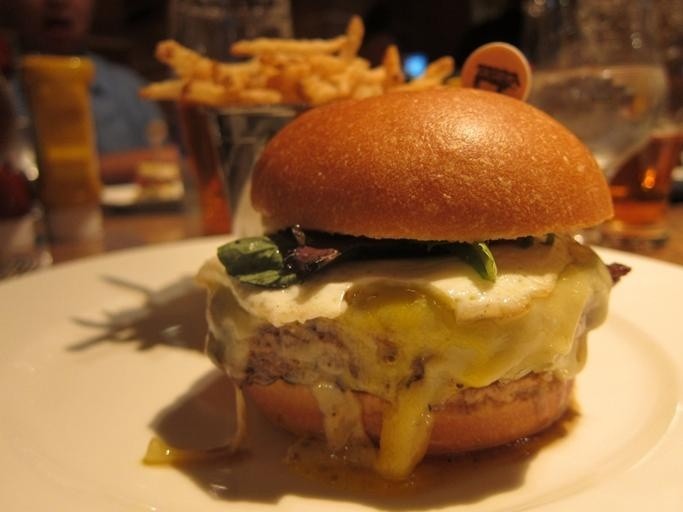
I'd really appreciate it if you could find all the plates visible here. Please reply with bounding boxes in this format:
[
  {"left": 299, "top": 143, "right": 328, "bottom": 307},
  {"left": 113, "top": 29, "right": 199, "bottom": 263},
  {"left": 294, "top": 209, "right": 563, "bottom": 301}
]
[
  {"left": 99, "top": 182, "right": 186, "bottom": 213},
  {"left": 1, "top": 235, "right": 681, "bottom": 510}
]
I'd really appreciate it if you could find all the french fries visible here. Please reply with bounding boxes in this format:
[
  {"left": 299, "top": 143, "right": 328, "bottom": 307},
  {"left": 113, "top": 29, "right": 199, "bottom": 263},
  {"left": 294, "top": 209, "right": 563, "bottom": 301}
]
[{"left": 138, "top": 14, "right": 456, "bottom": 108}]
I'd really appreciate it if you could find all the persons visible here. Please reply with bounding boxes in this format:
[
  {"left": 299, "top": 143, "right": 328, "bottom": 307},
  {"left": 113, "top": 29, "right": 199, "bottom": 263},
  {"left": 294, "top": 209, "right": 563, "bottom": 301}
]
[{"left": 7, "top": 0, "right": 167, "bottom": 151}]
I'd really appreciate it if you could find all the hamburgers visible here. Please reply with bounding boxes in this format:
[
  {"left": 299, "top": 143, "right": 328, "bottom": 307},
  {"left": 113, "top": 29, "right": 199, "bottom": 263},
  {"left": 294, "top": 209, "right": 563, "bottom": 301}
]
[{"left": 141, "top": 85, "right": 632, "bottom": 483}]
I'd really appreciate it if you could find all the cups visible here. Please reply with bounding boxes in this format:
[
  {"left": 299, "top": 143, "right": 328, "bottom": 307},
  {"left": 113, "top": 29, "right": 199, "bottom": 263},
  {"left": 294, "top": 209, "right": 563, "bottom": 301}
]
[{"left": 525, "top": 1, "right": 683, "bottom": 247}]
